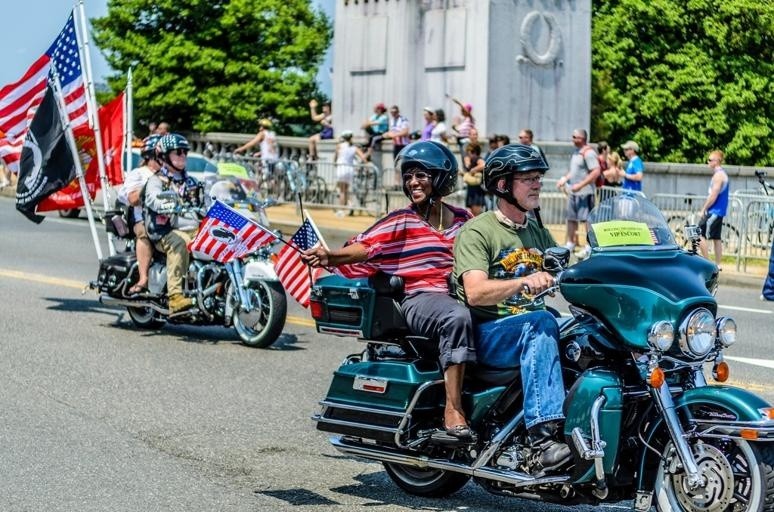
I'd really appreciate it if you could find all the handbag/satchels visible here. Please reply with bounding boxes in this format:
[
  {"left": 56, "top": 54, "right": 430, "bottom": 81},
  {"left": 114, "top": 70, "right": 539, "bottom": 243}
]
[{"left": 462, "top": 171, "right": 484, "bottom": 188}]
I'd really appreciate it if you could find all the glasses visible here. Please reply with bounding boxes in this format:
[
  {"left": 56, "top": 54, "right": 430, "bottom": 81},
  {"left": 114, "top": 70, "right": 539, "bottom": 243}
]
[
  {"left": 169, "top": 148, "right": 189, "bottom": 156},
  {"left": 400, "top": 172, "right": 433, "bottom": 184},
  {"left": 708, "top": 158, "right": 719, "bottom": 162},
  {"left": 506, "top": 173, "right": 546, "bottom": 185}
]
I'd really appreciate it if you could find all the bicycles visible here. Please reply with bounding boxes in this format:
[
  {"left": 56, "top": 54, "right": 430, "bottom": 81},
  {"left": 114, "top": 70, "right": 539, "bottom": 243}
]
[
  {"left": 659, "top": 170, "right": 774, "bottom": 257},
  {"left": 204, "top": 140, "right": 390, "bottom": 219}
]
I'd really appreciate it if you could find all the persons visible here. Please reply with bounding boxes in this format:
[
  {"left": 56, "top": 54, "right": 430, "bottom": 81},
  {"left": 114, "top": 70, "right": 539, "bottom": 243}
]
[
  {"left": 556, "top": 128, "right": 644, "bottom": 255},
  {"left": 332, "top": 132, "right": 369, "bottom": 217},
  {"left": 122, "top": 134, "right": 169, "bottom": 294},
  {"left": 697, "top": 148, "right": 730, "bottom": 271},
  {"left": 452, "top": 142, "right": 614, "bottom": 478},
  {"left": 360, "top": 94, "right": 476, "bottom": 189},
  {"left": 297, "top": 139, "right": 479, "bottom": 442},
  {"left": 131, "top": 121, "right": 171, "bottom": 147},
  {"left": 762, "top": 219, "right": 774, "bottom": 302},
  {"left": 459, "top": 128, "right": 542, "bottom": 224},
  {"left": 138, "top": 131, "right": 209, "bottom": 315},
  {"left": 307, "top": 99, "right": 334, "bottom": 161},
  {"left": 232, "top": 117, "right": 279, "bottom": 207}
]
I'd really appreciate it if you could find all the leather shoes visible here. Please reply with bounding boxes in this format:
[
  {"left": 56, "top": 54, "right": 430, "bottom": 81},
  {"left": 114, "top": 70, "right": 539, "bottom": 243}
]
[{"left": 441, "top": 415, "right": 472, "bottom": 438}]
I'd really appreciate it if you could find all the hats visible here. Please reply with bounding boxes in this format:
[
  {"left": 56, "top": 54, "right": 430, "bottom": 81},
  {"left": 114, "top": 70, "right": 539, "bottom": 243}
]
[
  {"left": 620, "top": 140, "right": 640, "bottom": 153},
  {"left": 453, "top": 97, "right": 475, "bottom": 119},
  {"left": 423, "top": 105, "right": 434, "bottom": 115}
]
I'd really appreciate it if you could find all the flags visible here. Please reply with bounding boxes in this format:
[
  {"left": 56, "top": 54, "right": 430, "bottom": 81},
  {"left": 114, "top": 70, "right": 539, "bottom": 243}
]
[
  {"left": 272, "top": 218, "right": 332, "bottom": 308},
  {"left": 0, "top": 1, "right": 128, "bottom": 225},
  {"left": 185, "top": 199, "right": 277, "bottom": 265}
]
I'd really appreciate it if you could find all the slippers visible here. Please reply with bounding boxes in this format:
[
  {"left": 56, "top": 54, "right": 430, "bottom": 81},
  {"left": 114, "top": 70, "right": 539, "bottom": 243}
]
[{"left": 129, "top": 282, "right": 148, "bottom": 294}]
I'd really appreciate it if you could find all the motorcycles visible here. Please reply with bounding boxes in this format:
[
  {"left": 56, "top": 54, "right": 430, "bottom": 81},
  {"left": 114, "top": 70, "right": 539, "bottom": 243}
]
[
  {"left": 82, "top": 151, "right": 288, "bottom": 349},
  {"left": 306, "top": 196, "right": 774, "bottom": 511}
]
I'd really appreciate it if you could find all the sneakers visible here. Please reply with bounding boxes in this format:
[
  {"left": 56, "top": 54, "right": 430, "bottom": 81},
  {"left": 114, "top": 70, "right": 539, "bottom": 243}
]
[
  {"left": 169, "top": 291, "right": 195, "bottom": 315},
  {"left": 334, "top": 208, "right": 357, "bottom": 218}
]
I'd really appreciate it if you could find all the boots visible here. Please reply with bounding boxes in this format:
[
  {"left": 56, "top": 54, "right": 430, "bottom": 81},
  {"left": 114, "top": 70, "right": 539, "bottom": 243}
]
[{"left": 523, "top": 417, "right": 587, "bottom": 481}]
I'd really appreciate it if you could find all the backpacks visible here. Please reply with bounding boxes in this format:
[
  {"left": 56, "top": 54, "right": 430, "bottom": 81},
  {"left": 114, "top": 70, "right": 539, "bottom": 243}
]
[{"left": 583, "top": 145, "right": 606, "bottom": 188}]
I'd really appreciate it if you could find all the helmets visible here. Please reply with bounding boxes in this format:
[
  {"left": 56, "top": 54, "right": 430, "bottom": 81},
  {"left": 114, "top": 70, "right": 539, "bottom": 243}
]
[
  {"left": 139, "top": 132, "right": 164, "bottom": 156},
  {"left": 482, "top": 141, "right": 551, "bottom": 190},
  {"left": 393, "top": 139, "right": 461, "bottom": 199},
  {"left": 257, "top": 117, "right": 274, "bottom": 129},
  {"left": 154, "top": 131, "right": 191, "bottom": 157},
  {"left": 340, "top": 130, "right": 354, "bottom": 141}
]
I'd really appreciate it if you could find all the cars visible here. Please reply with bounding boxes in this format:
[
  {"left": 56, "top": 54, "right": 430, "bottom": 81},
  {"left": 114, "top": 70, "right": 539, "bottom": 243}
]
[{"left": 58, "top": 145, "right": 217, "bottom": 220}]
